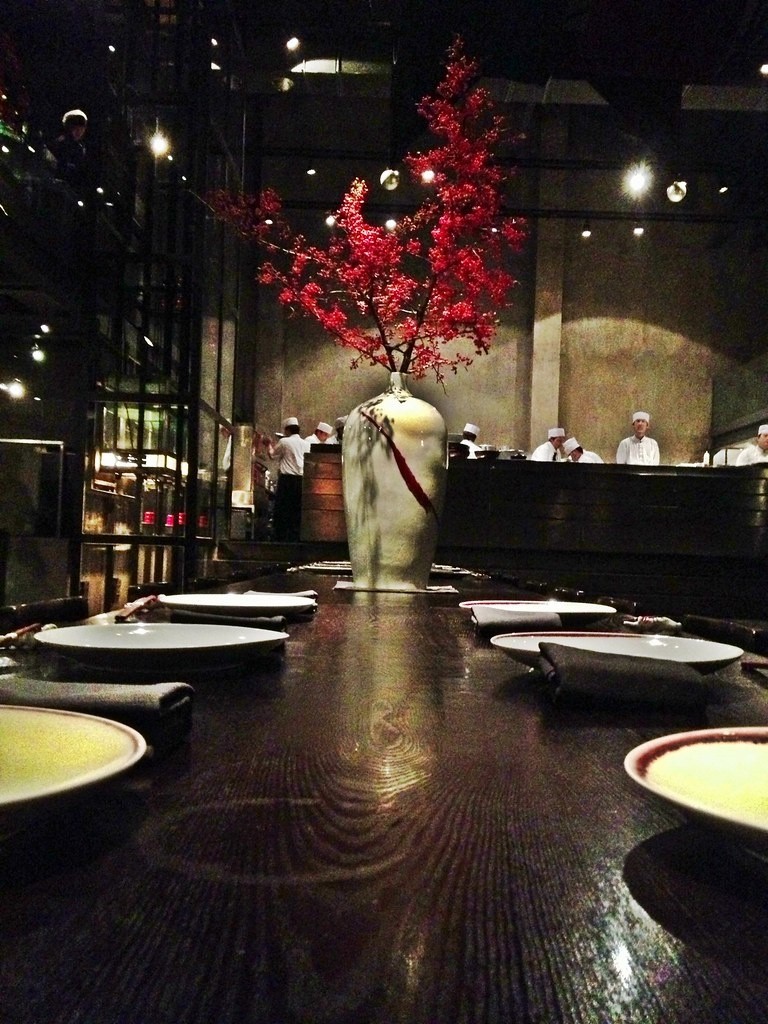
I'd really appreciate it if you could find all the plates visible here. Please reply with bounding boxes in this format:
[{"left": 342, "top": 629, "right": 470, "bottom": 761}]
[
  {"left": 430, "top": 563, "right": 470, "bottom": 575},
  {"left": 34, "top": 623, "right": 290, "bottom": 665},
  {"left": 303, "top": 559, "right": 351, "bottom": 571},
  {"left": 160, "top": 594, "right": 315, "bottom": 616},
  {"left": 0, "top": 703, "right": 147, "bottom": 804},
  {"left": 623, "top": 726, "right": 768, "bottom": 858},
  {"left": 490, "top": 632, "right": 744, "bottom": 678},
  {"left": 458, "top": 600, "right": 617, "bottom": 625}
]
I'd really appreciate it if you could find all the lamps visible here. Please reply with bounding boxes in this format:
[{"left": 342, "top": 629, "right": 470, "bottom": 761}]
[{"left": 581, "top": 218, "right": 592, "bottom": 238}]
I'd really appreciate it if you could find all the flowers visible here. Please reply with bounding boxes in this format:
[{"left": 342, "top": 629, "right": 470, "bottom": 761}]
[{"left": 208, "top": 35, "right": 529, "bottom": 398}]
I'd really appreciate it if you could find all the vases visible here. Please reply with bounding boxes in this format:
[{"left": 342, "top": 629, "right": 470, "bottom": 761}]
[{"left": 342, "top": 371, "right": 448, "bottom": 590}]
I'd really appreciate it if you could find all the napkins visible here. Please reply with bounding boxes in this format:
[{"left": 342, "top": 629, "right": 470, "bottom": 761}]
[
  {"left": 0, "top": 673, "right": 194, "bottom": 708},
  {"left": 537, "top": 642, "right": 712, "bottom": 705},
  {"left": 245, "top": 589, "right": 318, "bottom": 599},
  {"left": 469, "top": 606, "right": 562, "bottom": 636},
  {"left": 173, "top": 608, "right": 286, "bottom": 624}
]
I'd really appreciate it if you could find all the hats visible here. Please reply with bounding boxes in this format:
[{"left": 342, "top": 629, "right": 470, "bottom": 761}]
[
  {"left": 317, "top": 422, "right": 333, "bottom": 434},
  {"left": 334, "top": 415, "right": 348, "bottom": 428},
  {"left": 219, "top": 418, "right": 231, "bottom": 429},
  {"left": 547, "top": 428, "right": 565, "bottom": 440},
  {"left": 633, "top": 411, "right": 650, "bottom": 423},
  {"left": 281, "top": 417, "right": 299, "bottom": 426},
  {"left": 757, "top": 425, "right": 768, "bottom": 435},
  {"left": 561, "top": 437, "right": 580, "bottom": 455},
  {"left": 463, "top": 423, "right": 480, "bottom": 437}
]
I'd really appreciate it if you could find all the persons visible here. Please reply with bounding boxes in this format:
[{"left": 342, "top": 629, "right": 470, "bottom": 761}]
[
  {"left": 460, "top": 423, "right": 484, "bottom": 459},
  {"left": 531, "top": 428, "right": 565, "bottom": 462},
  {"left": 562, "top": 437, "right": 604, "bottom": 463},
  {"left": 266, "top": 417, "right": 311, "bottom": 541},
  {"left": 304, "top": 417, "right": 346, "bottom": 445},
  {"left": 616, "top": 412, "right": 660, "bottom": 465},
  {"left": 735, "top": 425, "right": 768, "bottom": 466},
  {"left": 218, "top": 418, "right": 232, "bottom": 518}
]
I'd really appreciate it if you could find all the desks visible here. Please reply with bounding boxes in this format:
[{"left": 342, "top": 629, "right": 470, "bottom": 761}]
[{"left": 0, "top": 558, "right": 768, "bottom": 1023}]
